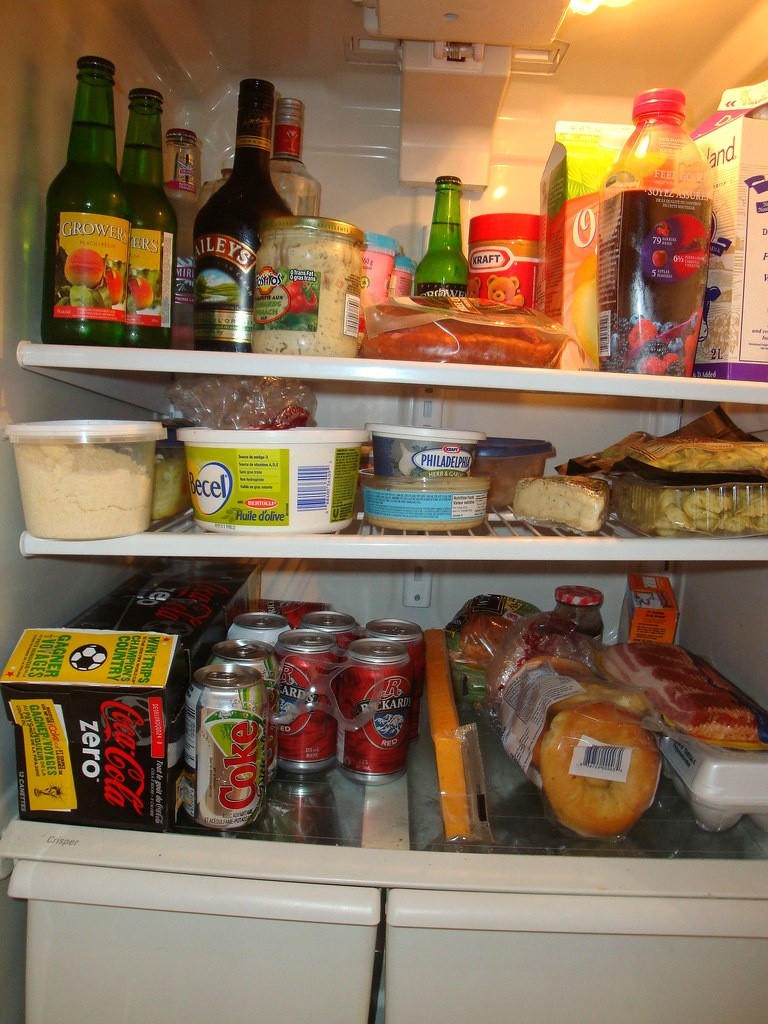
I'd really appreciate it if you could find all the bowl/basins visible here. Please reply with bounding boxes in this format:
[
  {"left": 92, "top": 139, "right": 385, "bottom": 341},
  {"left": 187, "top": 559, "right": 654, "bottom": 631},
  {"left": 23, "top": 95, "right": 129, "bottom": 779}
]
[{"left": 5, "top": 417, "right": 556, "bottom": 541}]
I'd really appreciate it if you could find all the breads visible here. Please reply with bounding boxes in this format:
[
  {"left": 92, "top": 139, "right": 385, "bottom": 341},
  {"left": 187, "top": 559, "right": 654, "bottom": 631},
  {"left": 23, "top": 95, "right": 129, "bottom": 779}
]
[
  {"left": 455, "top": 613, "right": 663, "bottom": 838},
  {"left": 511, "top": 476, "right": 610, "bottom": 531}
]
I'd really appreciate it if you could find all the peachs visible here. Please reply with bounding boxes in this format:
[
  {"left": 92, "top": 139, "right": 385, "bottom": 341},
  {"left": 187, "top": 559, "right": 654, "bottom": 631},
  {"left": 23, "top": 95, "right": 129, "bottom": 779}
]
[{"left": 65, "top": 248, "right": 154, "bottom": 310}]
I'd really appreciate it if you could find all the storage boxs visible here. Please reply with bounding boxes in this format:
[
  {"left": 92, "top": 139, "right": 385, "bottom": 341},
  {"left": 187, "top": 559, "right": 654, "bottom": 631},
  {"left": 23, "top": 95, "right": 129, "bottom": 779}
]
[
  {"left": 617, "top": 572, "right": 679, "bottom": 644},
  {"left": 0, "top": 560, "right": 265, "bottom": 832}
]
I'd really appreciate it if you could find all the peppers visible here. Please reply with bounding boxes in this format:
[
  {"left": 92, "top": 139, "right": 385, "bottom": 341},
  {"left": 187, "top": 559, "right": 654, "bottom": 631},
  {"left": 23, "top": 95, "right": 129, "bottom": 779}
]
[{"left": 276, "top": 269, "right": 318, "bottom": 312}]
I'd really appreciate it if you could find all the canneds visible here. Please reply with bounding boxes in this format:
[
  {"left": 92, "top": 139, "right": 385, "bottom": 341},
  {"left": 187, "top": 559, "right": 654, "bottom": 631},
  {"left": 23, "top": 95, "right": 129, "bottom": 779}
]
[
  {"left": 183, "top": 599, "right": 424, "bottom": 830},
  {"left": 466, "top": 211, "right": 542, "bottom": 305},
  {"left": 549, "top": 586, "right": 605, "bottom": 643},
  {"left": 250, "top": 215, "right": 366, "bottom": 362}
]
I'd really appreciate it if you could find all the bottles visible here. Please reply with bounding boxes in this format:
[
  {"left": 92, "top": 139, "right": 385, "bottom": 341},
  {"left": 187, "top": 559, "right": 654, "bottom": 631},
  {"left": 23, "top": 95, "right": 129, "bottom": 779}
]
[
  {"left": 41, "top": 54, "right": 130, "bottom": 346},
  {"left": 161, "top": 127, "right": 200, "bottom": 350},
  {"left": 552, "top": 584, "right": 604, "bottom": 645},
  {"left": 193, "top": 78, "right": 292, "bottom": 353},
  {"left": 415, "top": 175, "right": 469, "bottom": 297},
  {"left": 270, "top": 96, "right": 322, "bottom": 216},
  {"left": 598, "top": 87, "right": 713, "bottom": 378},
  {"left": 120, "top": 87, "right": 179, "bottom": 349}
]
